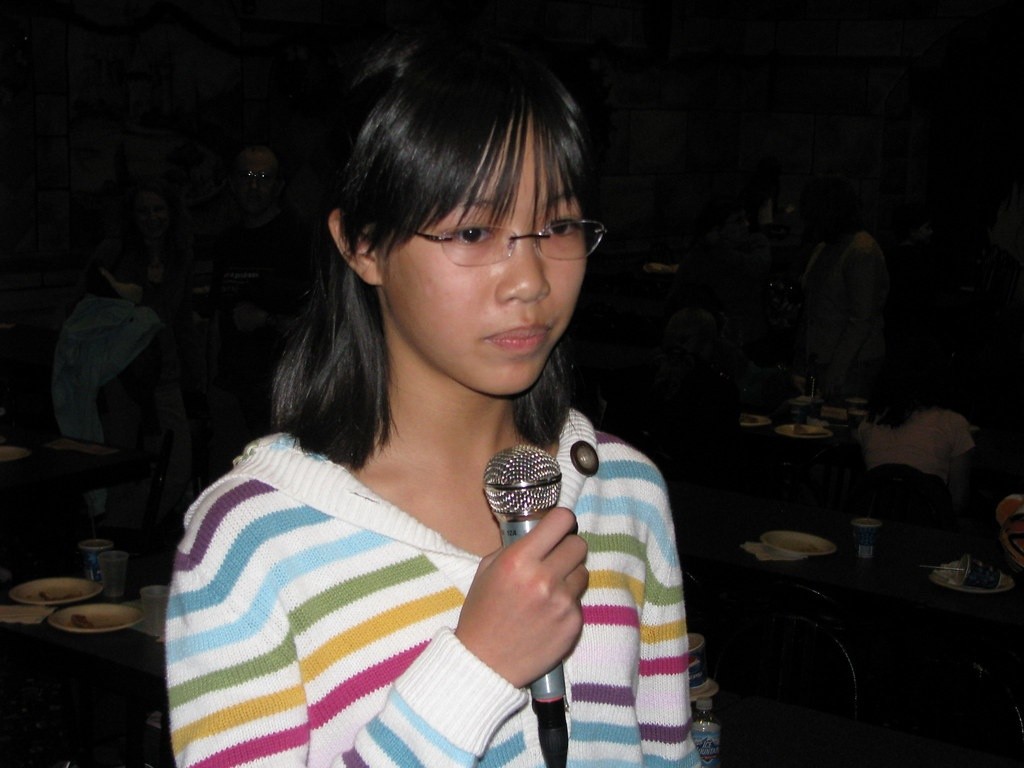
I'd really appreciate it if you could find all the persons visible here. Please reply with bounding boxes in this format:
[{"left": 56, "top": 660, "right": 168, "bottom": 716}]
[
  {"left": 786, "top": 172, "right": 890, "bottom": 405},
  {"left": 209, "top": 146, "right": 309, "bottom": 485},
  {"left": 859, "top": 341, "right": 977, "bottom": 489},
  {"left": 598, "top": 305, "right": 752, "bottom": 490},
  {"left": 165, "top": 39, "right": 698, "bottom": 768},
  {"left": 892, "top": 200, "right": 947, "bottom": 294},
  {"left": 87, "top": 177, "right": 199, "bottom": 553},
  {"left": 704, "top": 189, "right": 779, "bottom": 305}
]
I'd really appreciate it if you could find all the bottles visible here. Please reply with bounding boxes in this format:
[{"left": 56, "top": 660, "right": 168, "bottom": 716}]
[{"left": 691, "top": 697, "right": 721, "bottom": 768}]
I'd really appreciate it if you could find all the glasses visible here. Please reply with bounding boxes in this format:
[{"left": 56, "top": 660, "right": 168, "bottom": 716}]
[
  {"left": 235, "top": 168, "right": 271, "bottom": 188},
  {"left": 411, "top": 215, "right": 609, "bottom": 266},
  {"left": 725, "top": 214, "right": 746, "bottom": 226}
]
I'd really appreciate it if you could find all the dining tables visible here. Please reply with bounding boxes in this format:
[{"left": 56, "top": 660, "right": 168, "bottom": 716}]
[{"left": 2, "top": 261, "right": 1024, "bottom": 768}]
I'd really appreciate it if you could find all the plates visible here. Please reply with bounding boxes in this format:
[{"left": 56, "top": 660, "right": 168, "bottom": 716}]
[
  {"left": 689, "top": 677, "right": 718, "bottom": 702},
  {"left": 761, "top": 530, "right": 837, "bottom": 555},
  {"left": 9, "top": 577, "right": 103, "bottom": 605},
  {"left": 929, "top": 563, "right": 1015, "bottom": 593},
  {"left": 774, "top": 424, "right": 833, "bottom": 439},
  {"left": 820, "top": 406, "right": 846, "bottom": 421},
  {"left": 740, "top": 414, "right": 772, "bottom": 426},
  {"left": 0, "top": 446, "right": 31, "bottom": 462},
  {"left": 47, "top": 603, "right": 143, "bottom": 634}
]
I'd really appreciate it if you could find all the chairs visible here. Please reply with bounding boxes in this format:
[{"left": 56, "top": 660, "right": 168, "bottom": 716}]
[
  {"left": 905, "top": 653, "right": 1024, "bottom": 762},
  {"left": 858, "top": 464, "right": 957, "bottom": 533},
  {"left": 713, "top": 614, "right": 859, "bottom": 722}
]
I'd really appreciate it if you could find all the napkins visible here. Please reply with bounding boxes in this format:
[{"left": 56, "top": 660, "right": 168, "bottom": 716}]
[{"left": 739, "top": 539, "right": 808, "bottom": 562}]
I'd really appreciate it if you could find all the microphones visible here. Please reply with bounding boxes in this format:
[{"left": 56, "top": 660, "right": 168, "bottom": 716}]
[{"left": 482, "top": 442, "right": 569, "bottom": 767}]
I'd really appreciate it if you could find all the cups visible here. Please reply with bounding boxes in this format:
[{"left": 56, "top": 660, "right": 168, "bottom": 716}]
[
  {"left": 851, "top": 518, "right": 881, "bottom": 557},
  {"left": 847, "top": 398, "right": 869, "bottom": 431},
  {"left": 79, "top": 538, "right": 113, "bottom": 582},
  {"left": 687, "top": 632, "right": 708, "bottom": 689},
  {"left": 789, "top": 401, "right": 808, "bottom": 424},
  {"left": 809, "top": 399, "right": 824, "bottom": 418},
  {"left": 955, "top": 554, "right": 1002, "bottom": 591},
  {"left": 98, "top": 550, "right": 129, "bottom": 598},
  {"left": 139, "top": 585, "right": 168, "bottom": 638}
]
